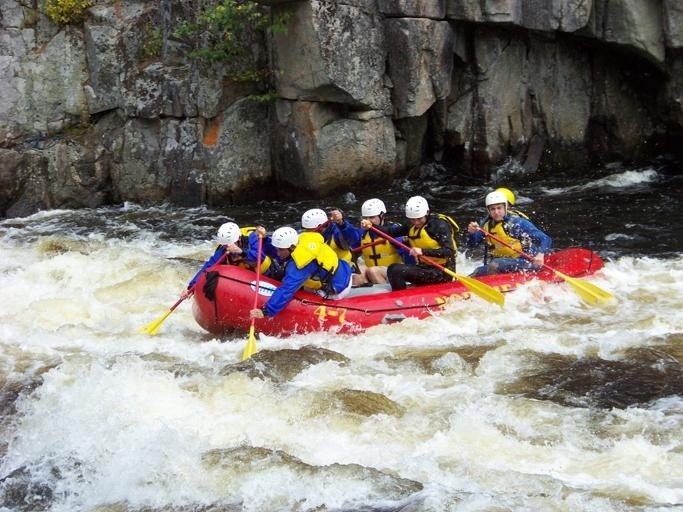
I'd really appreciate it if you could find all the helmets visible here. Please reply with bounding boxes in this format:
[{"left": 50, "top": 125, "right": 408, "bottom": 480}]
[
  {"left": 405, "top": 196, "right": 429, "bottom": 219},
  {"left": 495, "top": 188, "right": 515, "bottom": 206},
  {"left": 218, "top": 222, "right": 240, "bottom": 245},
  {"left": 361, "top": 199, "right": 386, "bottom": 217},
  {"left": 486, "top": 192, "right": 507, "bottom": 210},
  {"left": 271, "top": 227, "right": 298, "bottom": 248},
  {"left": 302, "top": 209, "right": 328, "bottom": 228}
]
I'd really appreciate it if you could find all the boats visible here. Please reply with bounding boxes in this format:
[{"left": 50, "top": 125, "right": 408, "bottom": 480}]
[{"left": 193, "top": 250, "right": 604, "bottom": 338}]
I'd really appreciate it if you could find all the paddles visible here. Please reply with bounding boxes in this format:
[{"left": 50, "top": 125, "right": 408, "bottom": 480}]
[
  {"left": 363, "top": 222, "right": 505, "bottom": 308},
  {"left": 243, "top": 226, "right": 265, "bottom": 360},
  {"left": 468, "top": 223, "right": 614, "bottom": 306},
  {"left": 139, "top": 250, "right": 231, "bottom": 335}
]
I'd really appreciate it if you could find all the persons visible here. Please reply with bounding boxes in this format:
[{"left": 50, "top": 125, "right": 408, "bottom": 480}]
[
  {"left": 360, "top": 195, "right": 459, "bottom": 293},
  {"left": 249, "top": 227, "right": 353, "bottom": 318},
  {"left": 301, "top": 208, "right": 357, "bottom": 273},
  {"left": 179, "top": 222, "right": 287, "bottom": 302},
  {"left": 496, "top": 187, "right": 532, "bottom": 223},
  {"left": 463, "top": 191, "right": 552, "bottom": 278},
  {"left": 330, "top": 199, "right": 416, "bottom": 287}
]
